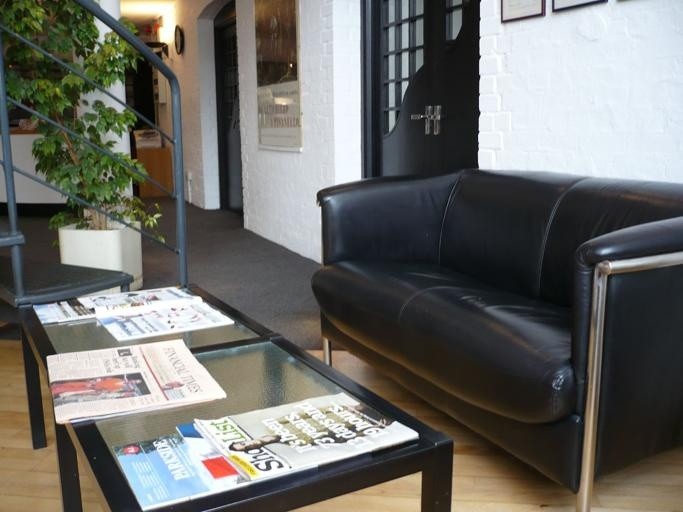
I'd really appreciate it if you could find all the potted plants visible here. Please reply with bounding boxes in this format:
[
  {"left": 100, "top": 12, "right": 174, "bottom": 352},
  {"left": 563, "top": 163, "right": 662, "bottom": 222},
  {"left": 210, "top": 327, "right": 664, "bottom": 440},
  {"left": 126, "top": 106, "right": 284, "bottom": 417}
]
[{"left": 0, "top": 0, "right": 166, "bottom": 294}]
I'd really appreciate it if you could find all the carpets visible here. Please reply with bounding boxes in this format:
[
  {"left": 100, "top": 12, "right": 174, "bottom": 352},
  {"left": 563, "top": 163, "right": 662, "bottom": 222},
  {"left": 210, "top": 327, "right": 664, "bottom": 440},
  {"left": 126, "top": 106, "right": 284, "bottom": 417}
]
[{"left": 0, "top": 339, "right": 683, "bottom": 512}]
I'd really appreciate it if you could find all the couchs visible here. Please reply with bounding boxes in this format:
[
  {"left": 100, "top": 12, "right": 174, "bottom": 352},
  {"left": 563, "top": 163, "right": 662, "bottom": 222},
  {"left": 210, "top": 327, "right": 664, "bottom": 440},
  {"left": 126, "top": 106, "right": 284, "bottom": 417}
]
[{"left": 311, "top": 168, "right": 683, "bottom": 512}]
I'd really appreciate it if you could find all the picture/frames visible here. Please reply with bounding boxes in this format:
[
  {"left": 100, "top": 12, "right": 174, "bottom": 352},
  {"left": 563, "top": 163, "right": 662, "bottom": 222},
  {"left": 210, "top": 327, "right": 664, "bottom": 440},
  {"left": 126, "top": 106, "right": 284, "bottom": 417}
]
[
  {"left": 552, "top": 0, "right": 608, "bottom": 12},
  {"left": 500, "top": 0, "right": 546, "bottom": 23}
]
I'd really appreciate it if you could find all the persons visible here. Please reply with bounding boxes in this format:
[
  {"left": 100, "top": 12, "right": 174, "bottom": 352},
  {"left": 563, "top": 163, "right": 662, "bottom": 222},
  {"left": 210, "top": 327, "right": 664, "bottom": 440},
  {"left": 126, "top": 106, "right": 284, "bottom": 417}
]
[
  {"left": 50, "top": 374, "right": 143, "bottom": 397},
  {"left": 229, "top": 402, "right": 393, "bottom": 452}
]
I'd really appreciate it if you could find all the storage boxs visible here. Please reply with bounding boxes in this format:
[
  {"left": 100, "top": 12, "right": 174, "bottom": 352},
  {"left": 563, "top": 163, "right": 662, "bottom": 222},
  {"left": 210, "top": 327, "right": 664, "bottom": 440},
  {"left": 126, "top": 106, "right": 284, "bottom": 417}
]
[{"left": 133, "top": 129, "right": 162, "bottom": 149}]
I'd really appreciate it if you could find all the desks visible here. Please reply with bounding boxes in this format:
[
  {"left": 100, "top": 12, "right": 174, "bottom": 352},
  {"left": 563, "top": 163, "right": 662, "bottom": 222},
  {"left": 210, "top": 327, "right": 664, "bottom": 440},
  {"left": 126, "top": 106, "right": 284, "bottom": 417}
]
[{"left": 136, "top": 147, "right": 173, "bottom": 198}]
